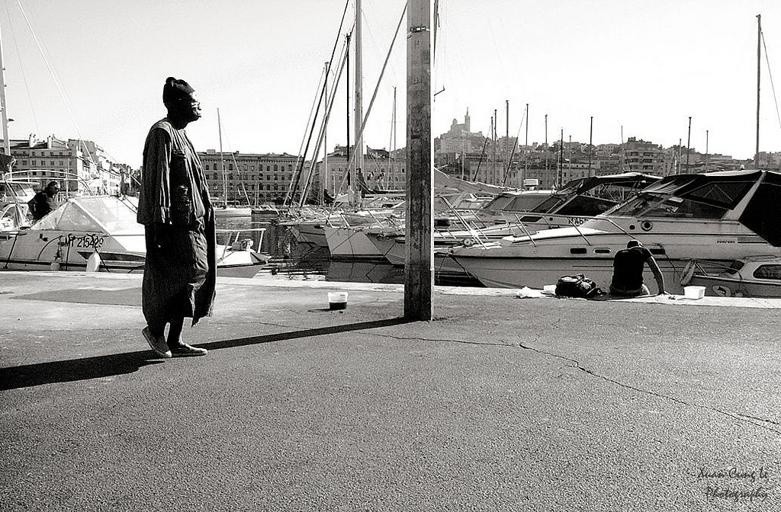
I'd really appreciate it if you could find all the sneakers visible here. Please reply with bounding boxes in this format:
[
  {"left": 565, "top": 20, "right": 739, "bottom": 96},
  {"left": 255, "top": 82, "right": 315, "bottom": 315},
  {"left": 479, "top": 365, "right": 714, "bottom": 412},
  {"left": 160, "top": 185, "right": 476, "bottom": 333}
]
[
  {"left": 142, "top": 326, "right": 172, "bottom": 358},
  {"left": 171, "top": 343, "right": 208, "bottom": 358}
]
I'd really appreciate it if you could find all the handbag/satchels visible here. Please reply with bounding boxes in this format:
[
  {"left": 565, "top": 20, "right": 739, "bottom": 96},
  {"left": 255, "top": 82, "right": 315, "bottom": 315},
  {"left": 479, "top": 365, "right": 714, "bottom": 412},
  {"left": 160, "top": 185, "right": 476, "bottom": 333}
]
[{"left": 555, "top": 273, "right": 608, "bottom": 299}]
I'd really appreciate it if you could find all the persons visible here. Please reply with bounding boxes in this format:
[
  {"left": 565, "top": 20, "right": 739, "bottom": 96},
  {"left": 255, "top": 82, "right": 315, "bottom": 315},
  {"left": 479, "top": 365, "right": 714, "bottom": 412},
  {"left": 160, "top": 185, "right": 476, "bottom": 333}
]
[
  {"left": 367, "top": 171, "right": 385, "bottom": 190},
  {"left": 609, "top": 240, "right": 669, "bottom": 299},
  {"left": 324, "top": 189, "right": 335, "bottom": 203},
  {"left": 28, "top": 181, "right": 61, "bottom": 224},
  {"left": 136, "top": 77, "right": 216, "bottom": 358}
]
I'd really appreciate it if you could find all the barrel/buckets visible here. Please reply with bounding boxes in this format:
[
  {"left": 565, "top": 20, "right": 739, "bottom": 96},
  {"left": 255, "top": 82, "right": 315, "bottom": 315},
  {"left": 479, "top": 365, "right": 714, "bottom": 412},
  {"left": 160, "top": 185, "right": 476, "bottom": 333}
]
[{"left": 327, "top": 292, "right": 348, "bottom": 310}]
